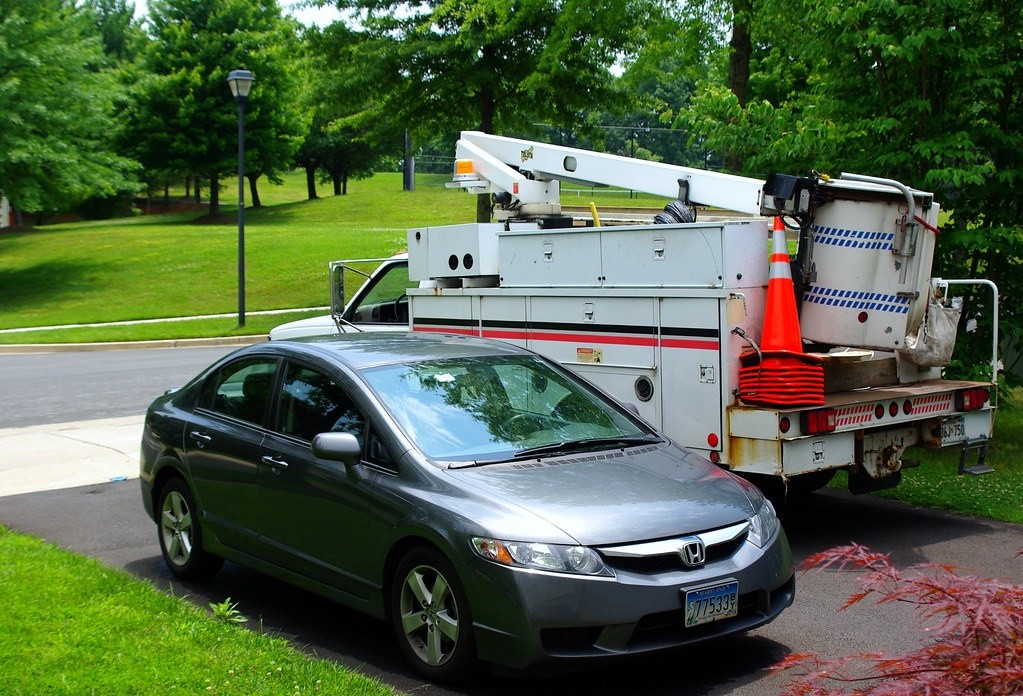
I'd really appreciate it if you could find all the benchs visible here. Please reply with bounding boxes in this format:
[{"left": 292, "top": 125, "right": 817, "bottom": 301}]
[{"left": 225, "top": 372, "right": 274, "bottom": 425}]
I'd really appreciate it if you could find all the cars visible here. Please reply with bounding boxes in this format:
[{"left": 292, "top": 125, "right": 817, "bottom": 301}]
[{"left": 138, "top": 329, "right": 794, "bottom": 690}]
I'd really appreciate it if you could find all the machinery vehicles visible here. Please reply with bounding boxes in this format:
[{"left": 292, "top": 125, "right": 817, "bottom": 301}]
[{"left": 267, "top": 131, "right": 1000, "bottom": 503}]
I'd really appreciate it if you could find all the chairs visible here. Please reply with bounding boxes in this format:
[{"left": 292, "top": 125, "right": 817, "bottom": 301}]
[{"left": 284, "top": 373, "right": 343, "bottom": 441}]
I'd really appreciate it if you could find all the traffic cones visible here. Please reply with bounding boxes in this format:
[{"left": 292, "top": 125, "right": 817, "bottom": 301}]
[{"left": 737, "top": 216, "right": 825, "bottom": 408}]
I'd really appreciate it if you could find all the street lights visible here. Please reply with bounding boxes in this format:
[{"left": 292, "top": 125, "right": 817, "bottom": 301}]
[{"left": 227, "top": 65, "right": 255, "bottom": 329}]
